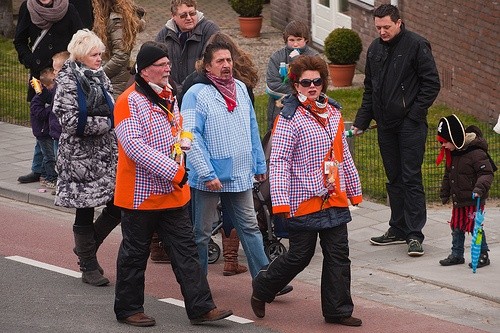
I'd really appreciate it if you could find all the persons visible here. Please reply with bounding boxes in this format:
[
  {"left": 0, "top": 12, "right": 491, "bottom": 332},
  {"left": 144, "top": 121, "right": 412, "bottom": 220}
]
[
  {"left": 113, "top": 39, "right": 233, "bottom": 326},
  {"left": 13, "top": 1, "right": 321, "bottom": 296},
  {"left": 351, "top": 4, "right": 441, "bottom": 256},
  {"left": 434, "top": 114, "right": 498, "bottom": 269},
  {"left": 250, "top": 54, "right": 363, "bottom": 327}
]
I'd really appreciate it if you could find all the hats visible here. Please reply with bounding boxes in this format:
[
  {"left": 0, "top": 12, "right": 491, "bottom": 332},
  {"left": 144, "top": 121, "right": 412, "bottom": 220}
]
[
  {"left": 436, "top": 119, "right": 453, "bottom": 144},
  {"left": 137, "top": 45, "right": 170, "bottom": 74}
]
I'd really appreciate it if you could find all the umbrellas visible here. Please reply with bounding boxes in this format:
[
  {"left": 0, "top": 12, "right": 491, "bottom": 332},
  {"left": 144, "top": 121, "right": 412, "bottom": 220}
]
[{"left": 470, "top": 193, "right": 485, "bottom": 273}]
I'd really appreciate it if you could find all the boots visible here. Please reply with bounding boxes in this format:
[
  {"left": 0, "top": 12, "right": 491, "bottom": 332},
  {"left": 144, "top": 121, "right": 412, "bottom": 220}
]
[
  {"left": 73, "top": 207, "right": 121, "bottom": 275},
  {"left": 220, "top": 227, "right": 247, "bottom": 275},
  {"left": 73, "top": 223, "right": 110, "bottom": 286}
]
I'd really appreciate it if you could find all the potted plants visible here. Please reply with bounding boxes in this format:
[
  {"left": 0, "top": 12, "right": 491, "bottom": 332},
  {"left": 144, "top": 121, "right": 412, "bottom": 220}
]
[
  {"left": 322, "top": 28, "right": 362, "bottom": 86},
  {"left": 229, "top": 0, "right": 264, "bottom": 37}
]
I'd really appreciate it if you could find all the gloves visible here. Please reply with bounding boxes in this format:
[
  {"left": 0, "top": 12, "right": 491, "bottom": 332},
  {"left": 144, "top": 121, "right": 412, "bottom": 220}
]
[
  {"left": 178, "top": 167, "right": 190, "bottom": 188},
  {"left": 92, "top": 104, "right": 111, "bottom": 117}
]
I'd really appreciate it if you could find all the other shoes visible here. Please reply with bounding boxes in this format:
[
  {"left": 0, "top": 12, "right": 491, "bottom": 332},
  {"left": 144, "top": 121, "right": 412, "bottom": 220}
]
[
  {"left": 251, "top": 293, "right": 265, "bottom": 318},
  {"left": 325, "top": 315, "right": 362, "bottom": 326},
  {"left": 190, "top": 307, "right": 233, "bottom": 324},
  {"left": 276, "top": 285, "right": 293, "bottom": 296},
  {"left": 150, "top": 238, "right": 171, "bottom": 261},
  {"left": 117, "top": 312, "right": 155, "bottom": 327},
  {"left": 40, "top": 177, "right": 56, "bottom": 189},
  {"left": 18, "top": 172, "right": 41, "bottom": 183}
]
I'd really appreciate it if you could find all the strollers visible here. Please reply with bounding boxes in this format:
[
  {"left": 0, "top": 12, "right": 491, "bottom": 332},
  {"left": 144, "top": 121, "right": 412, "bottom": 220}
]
[{"left": 206, "top": 177, "right": 287, "bottom": 266}]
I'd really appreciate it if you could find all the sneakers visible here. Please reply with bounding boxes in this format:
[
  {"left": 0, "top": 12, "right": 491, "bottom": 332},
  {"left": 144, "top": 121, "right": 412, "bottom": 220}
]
[
  {"left": 369, "top": 231, "right": 406, "bottom": 245},
  {"left": 407, "top": 239, "right": 424, "bottom": 256},
  {"left": 468, "top": 252, "right": 490, "bottom": 268},
  {"left": 439, "top": 254, "right": 465, "bottom": 266}
]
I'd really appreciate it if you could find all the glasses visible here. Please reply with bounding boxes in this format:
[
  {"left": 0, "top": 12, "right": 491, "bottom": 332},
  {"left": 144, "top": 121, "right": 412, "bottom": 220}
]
[
  {"left": 151, "top": 63, "right": 173, "bottom": 68},
  {"left": 175, "top": 11, "right": 197, "bottom": 19},
  {"left": 296, "top": 78, "right": 323, "bottom": 87}
]
